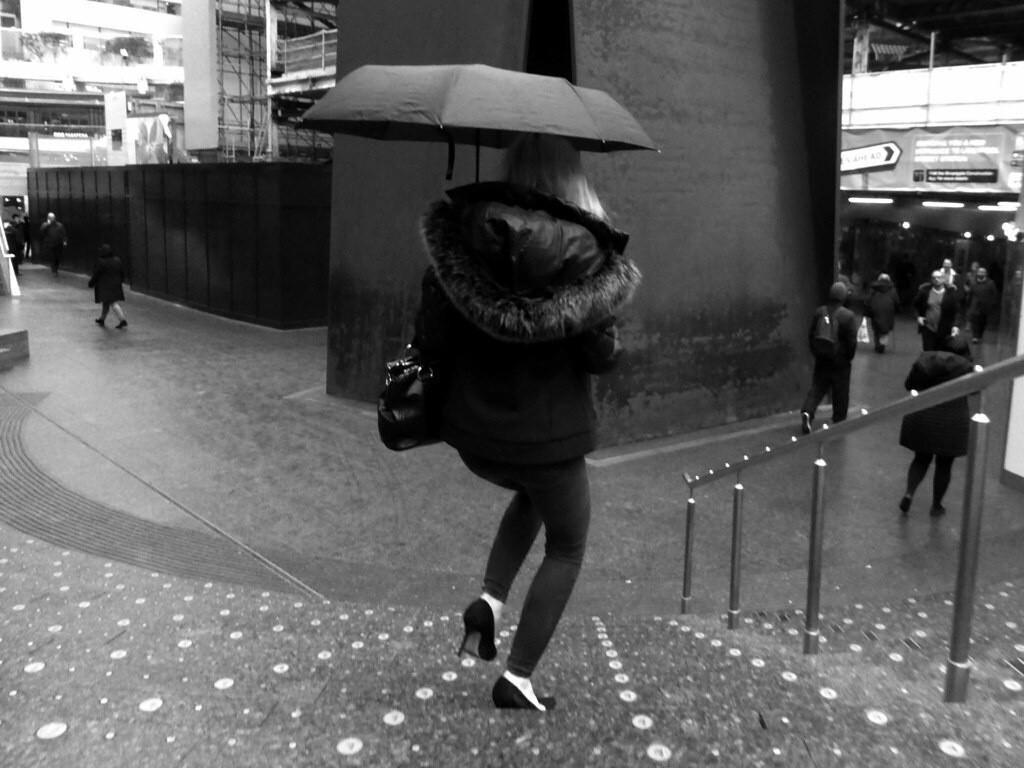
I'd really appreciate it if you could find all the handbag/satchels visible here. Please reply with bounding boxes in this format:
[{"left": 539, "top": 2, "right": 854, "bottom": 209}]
[
  {"left": 377, "top": 353, "right": 443, "bottom": 451},
  {"left": 856, "top": 316, "right": 869, "bottom": 343}
]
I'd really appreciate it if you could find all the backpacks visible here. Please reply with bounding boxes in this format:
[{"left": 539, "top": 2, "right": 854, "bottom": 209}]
[{"left": 812, "top": 305, "right": 846, "bottom": 354}]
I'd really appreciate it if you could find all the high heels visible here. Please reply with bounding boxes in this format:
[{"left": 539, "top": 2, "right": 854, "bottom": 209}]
[
  {"left": 493, "top": 675, "right": 556, "bottom": 712},
  {"left": 458, "top": 598, "right": 497, "bottom": 660}
]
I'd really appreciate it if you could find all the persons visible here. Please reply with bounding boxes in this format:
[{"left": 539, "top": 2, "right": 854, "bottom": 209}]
[
  {"left": 898, "top": 335, "right": 981, "bottom": 517},
  {"left": 86, "top": 245, "right": 128, "bottom": 330},
  {"left": 897, "top": 254, "right": 917, "bottom": 307},
  {"left": 801, "top": 274, "right": 861, "bottom": 434},
  {"left": 4, "top": 213, "right": 32, "bottom": 278},
  {"left": 916, "top": 258, "right": 997, "bottom": 350},
  {"left": 41, "top": 212, "right": 68, "bottom": 277},
  {"left": 416, "top": 134, "right": 642, "bottom": 716},
  {"left": 1005, "top": 232, "right": 1024, "bottom": 339},
  {"left": 864, "top": 272, "right": 901, "bottom": 352}
]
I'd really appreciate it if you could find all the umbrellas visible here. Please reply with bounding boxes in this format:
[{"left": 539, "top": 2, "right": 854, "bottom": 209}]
[{"left": 298, "top": 62, "right": 661, "bottom": 184}]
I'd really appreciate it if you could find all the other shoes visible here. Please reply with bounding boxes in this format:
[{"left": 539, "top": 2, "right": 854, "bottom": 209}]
[
  {"left": 115, "top": 320, "right": 128, "bottom": 328},
  {"left": 802, "top": 412, "right": 811, "bottom": 434},
  {"left": 931, "top": 506, "right": 945, "bottom": 516},
  {"left": 899, "top": 497, "right": 911, "bottom": 512},
  {"left": 96, "top": 319, "right": 105, "bottom": 325}
]
